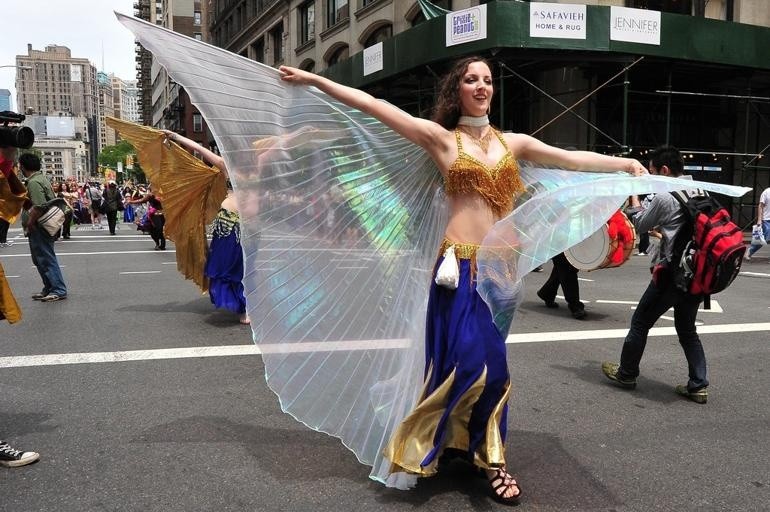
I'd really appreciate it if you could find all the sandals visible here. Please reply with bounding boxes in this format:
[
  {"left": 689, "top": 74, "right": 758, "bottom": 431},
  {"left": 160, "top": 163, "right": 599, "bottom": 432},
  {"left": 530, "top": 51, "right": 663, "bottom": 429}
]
[
  {"left": 572, "top": 306, "right": 585, "bottom": 321},
  {"left": 537, "top": 291, "right": 559, "bottom": 309},
  {"left": 486, "top": 464, "right": 523, "bottom": 502}
]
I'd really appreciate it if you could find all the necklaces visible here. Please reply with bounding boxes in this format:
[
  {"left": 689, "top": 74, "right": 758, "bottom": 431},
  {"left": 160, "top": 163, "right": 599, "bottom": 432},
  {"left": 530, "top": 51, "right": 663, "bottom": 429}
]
[{"left": 458, "top": 126, "right": 492, "bottom": 155}]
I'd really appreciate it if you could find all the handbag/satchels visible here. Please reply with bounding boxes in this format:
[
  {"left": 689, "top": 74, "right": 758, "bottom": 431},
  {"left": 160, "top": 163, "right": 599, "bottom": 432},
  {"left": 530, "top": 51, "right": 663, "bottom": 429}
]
[
  {"left": 29, "top": 181, "right": 66, "bottom": 237},
  {"left": 99, "top": 200, "right": 118, "bottom": 215},
  {"left": 88, "top": 189, "right": 102, "bottom": 210},
  {"left": 752, "top": 223, "right": 767, "bottom": 246}
]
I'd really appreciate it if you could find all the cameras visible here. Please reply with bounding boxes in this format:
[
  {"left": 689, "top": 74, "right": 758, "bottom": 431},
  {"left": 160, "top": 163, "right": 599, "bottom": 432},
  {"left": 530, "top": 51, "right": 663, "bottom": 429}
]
[
  {"left": 603, "top": 145, "right": 650, "bottom": 200},
  {"left": 1, "top": 110, "right": 34, "bottom": 149}
]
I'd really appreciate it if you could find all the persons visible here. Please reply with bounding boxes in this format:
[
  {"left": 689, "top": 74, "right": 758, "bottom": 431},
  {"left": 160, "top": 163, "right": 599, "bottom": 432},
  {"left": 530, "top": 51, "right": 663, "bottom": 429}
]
[
  {"left": 50, "top": 174, "right": 167, "bottom": 252},
  {"left": 533, "top": 266, "right": 544, "bottom": 272},
  {"left": 0, "top": 147, "right": 40, "bottom": 467},
  {"left": 18, "top": 153, "right": 68, "bottom": 303},
  {"left": 537, "top": 252, "right": 589, "bottom": 320},
  {"left": 601, "top": 144, "right": 712, "bottom": 404},
  {"left": 158, "top": 124, "right": 316, "bottom": 325},
  {"left": 279, "top": 55, "right": 649, "bottom": 505},
  {"left": 260, "top": 179, "right": 361, "bottom": 248},
  {"left": 637, "top": 193, "right": 655, "bottom": 256},
  {"left": 743, "top": 187, "right": 770, "bottom": 262}
]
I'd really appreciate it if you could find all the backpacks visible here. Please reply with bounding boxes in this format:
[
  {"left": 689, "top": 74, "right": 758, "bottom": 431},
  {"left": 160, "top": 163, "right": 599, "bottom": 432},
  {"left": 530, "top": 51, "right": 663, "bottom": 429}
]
[{"left": 650, "top": 189, "right": 748, "bottom": 309}]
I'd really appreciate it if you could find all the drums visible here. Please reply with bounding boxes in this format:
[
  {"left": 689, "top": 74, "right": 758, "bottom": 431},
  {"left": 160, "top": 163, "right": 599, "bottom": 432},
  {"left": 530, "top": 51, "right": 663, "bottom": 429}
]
[{"left": 565, "top": 209, "right": 637, "bottom": 272}]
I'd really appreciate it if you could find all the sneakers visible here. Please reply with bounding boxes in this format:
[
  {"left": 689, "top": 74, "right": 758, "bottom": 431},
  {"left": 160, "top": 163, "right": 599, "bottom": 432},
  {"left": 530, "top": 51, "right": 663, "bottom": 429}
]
[
  {"left": 42, "top": 294, "right": 68, "bottom": 302},
  {"left": 676, "top": 385, "right": 708, "bottom": 404},
  {"left": 602, "top": 361, "right": 638, "bottom": 388},
  {"left": 2, "top": 441, "right": 41, "bottom": 468},
  {"left": 33, "top": 292, "right": 48, "bottom": 300}
]
